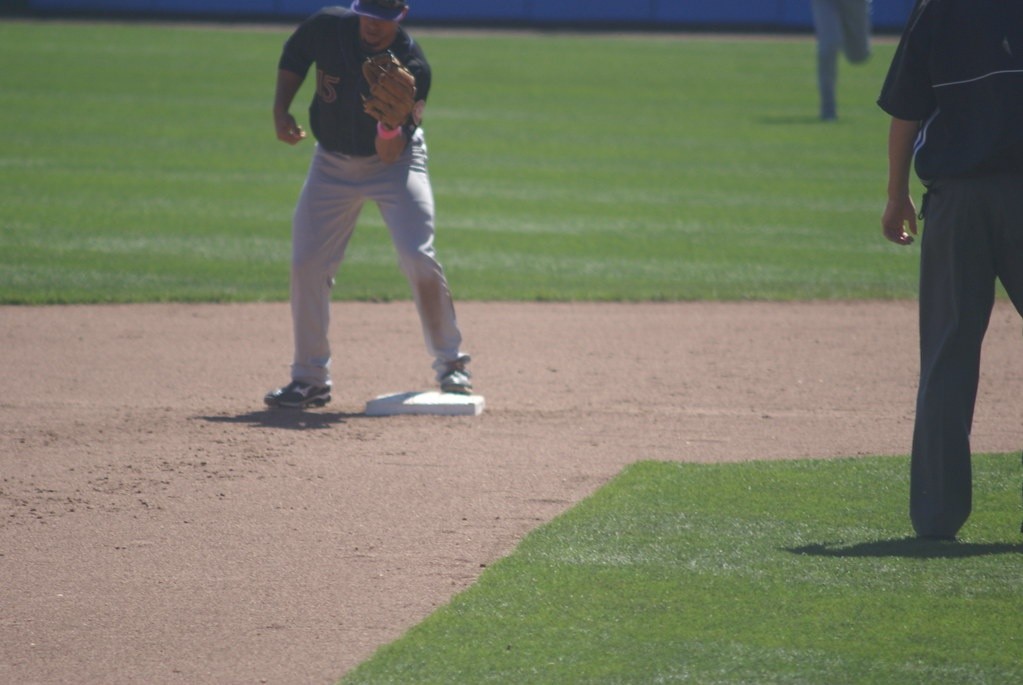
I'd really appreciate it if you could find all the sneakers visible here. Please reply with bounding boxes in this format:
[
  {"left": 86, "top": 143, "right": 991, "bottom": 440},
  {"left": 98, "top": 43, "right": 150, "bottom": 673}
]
[
  {"left": 441, "top": 357, "right": 473, "bottom": 394},
  {"left": 263, "top": 380, "right": 333, "bottom": 408}
]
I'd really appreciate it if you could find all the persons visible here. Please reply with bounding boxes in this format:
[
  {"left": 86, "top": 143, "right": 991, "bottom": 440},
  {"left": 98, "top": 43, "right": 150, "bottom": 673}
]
[
  {"left": 876, "top": 0, "right": 1023, "bottom": 542},
  {"left": 810, "top": 0, "right": 872, "bottom": 118},
  {"left": 261, "top": 0, "right": 473, "bottom": 408}
]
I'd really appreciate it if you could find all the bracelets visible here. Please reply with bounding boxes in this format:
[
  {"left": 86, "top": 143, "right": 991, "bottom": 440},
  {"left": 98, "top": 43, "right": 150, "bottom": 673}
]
[{"left": 376, "top": 123, "right": 403, "bottom": 140}]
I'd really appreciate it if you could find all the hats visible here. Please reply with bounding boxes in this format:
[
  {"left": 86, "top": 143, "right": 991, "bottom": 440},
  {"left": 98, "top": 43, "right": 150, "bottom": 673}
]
[{"left": 351, "top": 0, "right": 406, "bottom": 20}]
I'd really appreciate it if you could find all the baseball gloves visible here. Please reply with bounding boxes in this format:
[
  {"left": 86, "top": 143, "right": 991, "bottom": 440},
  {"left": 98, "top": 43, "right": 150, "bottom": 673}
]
[{"left": 359, "top": 48, "right": 419, "bottom": 130}]
